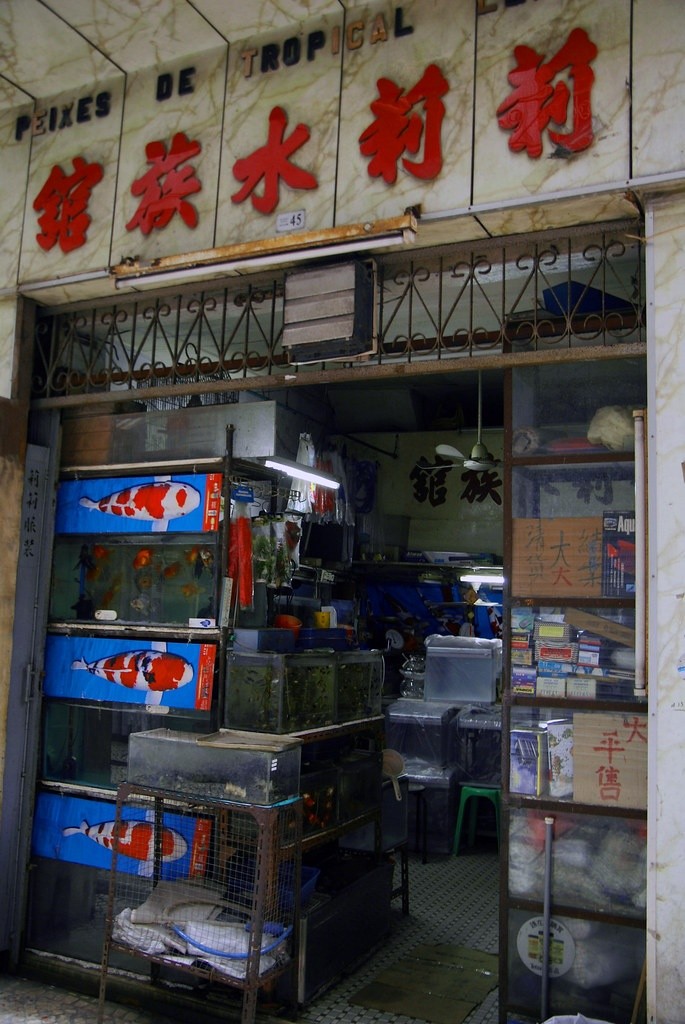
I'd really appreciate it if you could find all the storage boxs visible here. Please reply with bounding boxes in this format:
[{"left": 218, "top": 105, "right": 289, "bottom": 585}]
[
  {"left": 385, "top": 700, "right": 461, "bottom": 770},
  {"left": 232, "top": 858, "right": 320, "bottom": 909},
  {"left": 458, "top": 713, "right": 501, "bottom": 782},
  {"left": 424, "top": 643, "right": 497, "bottom": 706},
  {"left": 403, "top": 768, "right": 457, "bottom": 854},
  {"left": 126, "top": 728, "right": 305, "bottom": 806},
  {"left": 225, "top": 650, "right": 382, "bottom": 734},
  {"left": 48, "top": 531, "right": 222, "bottom": 627},
  {"left": 339, "top": 775, "right": 408, "bottom": 852}
]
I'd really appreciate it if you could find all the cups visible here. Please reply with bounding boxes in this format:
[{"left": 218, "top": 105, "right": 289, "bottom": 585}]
[{"left": 314, "top": 611, "right": 330, "bottom": 628}]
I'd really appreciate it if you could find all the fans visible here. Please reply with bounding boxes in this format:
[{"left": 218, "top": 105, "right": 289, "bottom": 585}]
[{"left": 415, "top": 370, "right": 504, "bottom": 471}]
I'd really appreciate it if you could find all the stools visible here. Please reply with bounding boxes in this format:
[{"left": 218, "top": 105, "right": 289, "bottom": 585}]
[
  {"left": 409, "top": 783, "right": 428, "bottom": 865},
  {"left": 451, "top": 786, "right": 502, "bottom": 863}
]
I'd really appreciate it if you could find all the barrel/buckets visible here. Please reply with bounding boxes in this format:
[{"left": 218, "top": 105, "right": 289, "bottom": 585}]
[{"left": 275, "top": 614, "right": 302, "bottom": 639}]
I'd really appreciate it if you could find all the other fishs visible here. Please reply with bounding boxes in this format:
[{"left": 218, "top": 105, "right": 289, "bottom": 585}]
[
  {"left": 288, "top": 786, "right": 336, "bottom": 833},
  {"left": 229, "top": 662, "right": 383, "bottom": 736},
  {"left": 69, "top": 541, "right": 216, "bottom": 622},
  {"left": 68, "top": 641, "right": 195, "bottom": 705},
  {"left": 79, "top": 475, "right": 204, "bottom": 532},
  {"left": 364, "top": 584, "right": 503, "bottom": 655},
  {"left": 62, "top": 809, "right": 188, "bottom": 878}
]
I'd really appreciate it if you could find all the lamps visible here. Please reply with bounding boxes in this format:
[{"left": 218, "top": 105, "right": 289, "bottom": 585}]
[
  {"left": 110, "top": 215, "right": 418, "bottom": 290},
  {"left": 259, "top": 456, "right": 342, "bottom": 490},
  {"left": 632, "top": 409, "right": 648, "bottom": 697}
]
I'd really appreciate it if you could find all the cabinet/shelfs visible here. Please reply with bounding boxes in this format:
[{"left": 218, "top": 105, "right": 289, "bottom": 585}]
[
  {"left": 219, "top": 714, "right": 385, "bottom": 922},
  {"left": 97, "top": 781, "right": 303, "bottom": 1024},
  {"left": 499, "top": 317, "right": 647, "bottom": 1024}
]
